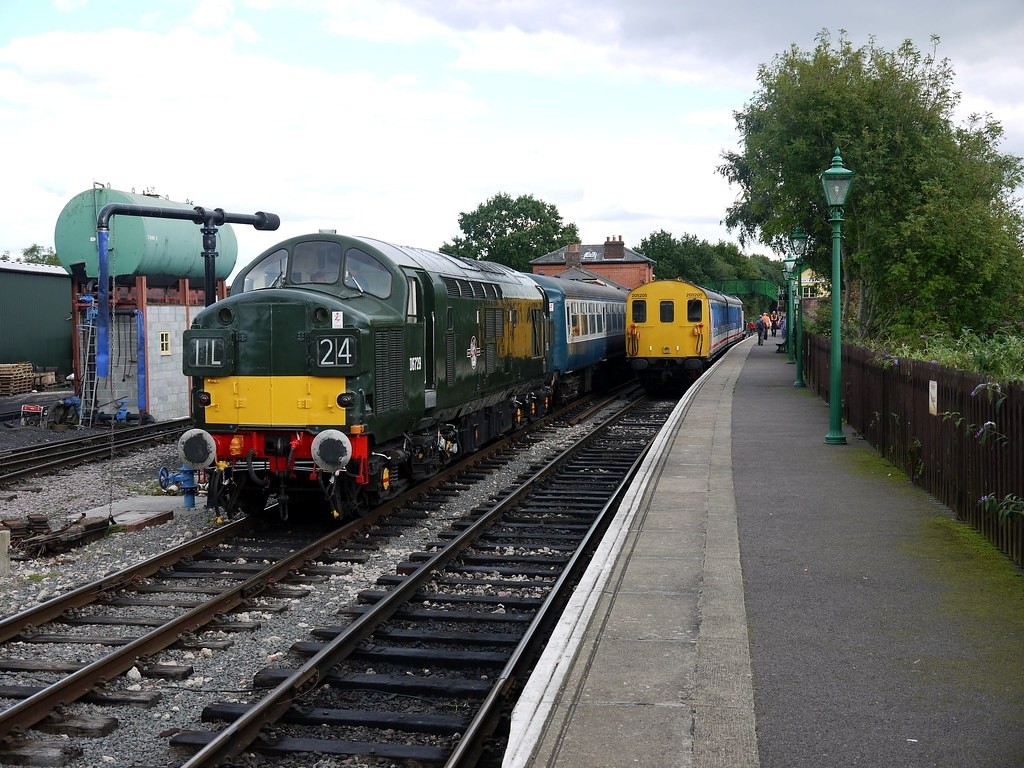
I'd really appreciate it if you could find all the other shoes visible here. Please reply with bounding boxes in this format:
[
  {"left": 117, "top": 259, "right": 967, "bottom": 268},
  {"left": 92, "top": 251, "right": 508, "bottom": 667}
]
[
  {"left": 758, "top": 343, "right": 760, "bottom": 345},
  {"left": 761, "top": 344, "right": 763, "bottom": 346}
]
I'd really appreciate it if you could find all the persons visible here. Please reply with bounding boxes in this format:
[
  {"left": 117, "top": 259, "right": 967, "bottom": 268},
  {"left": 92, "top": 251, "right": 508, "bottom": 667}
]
[
  {"left": 756, "top": 315, "right": 767, "bottom": 346},
  {"left": 762, "top": 313, "right": 771, "bottom": 340},
  {"left": 749, "top": 321, "right": 755, "bottom": 335},
  {"left": 771, "top": 311, "right": 778, "bottom": 337}
]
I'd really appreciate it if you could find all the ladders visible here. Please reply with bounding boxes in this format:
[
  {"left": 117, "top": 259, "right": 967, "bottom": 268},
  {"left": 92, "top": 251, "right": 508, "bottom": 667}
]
[{"left": 76, "top": 315, "right": 100, "bottom": 429}]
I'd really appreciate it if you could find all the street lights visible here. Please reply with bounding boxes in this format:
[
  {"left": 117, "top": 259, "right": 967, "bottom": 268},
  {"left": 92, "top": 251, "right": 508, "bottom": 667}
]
[
  {"left": 781, "top": 267, "right": 788, "bottom": 353},
  {"left": 786, "top": 253, "right": 795, "bottom": 364},
  {"left": 819, "top": 147, "right": 856, "bottom": 445},
  {"left": 793, "top": 224, "right": 810, "bottom": 388}
]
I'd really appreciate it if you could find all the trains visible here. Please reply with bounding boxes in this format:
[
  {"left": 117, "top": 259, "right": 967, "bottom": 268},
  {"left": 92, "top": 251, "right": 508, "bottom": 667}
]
[
  {"left": 625, "top": 278, "right": 747, "bottom": 394},
  {"left": 183, "top": 228, "right": 633, "bottom": 520}
]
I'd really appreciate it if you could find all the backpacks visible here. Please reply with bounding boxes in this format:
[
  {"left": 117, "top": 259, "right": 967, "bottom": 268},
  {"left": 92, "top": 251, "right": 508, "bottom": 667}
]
[{"left": 757, "top": 319, "right": 764, "bottom": 329}]
